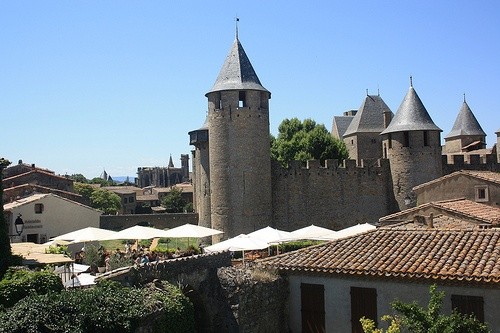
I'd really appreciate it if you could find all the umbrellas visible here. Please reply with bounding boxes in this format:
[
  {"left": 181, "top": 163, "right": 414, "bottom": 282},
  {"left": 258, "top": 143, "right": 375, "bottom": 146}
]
[
  {"left": 52, "top": 261, "right": 91, "bottom": 276},
  {"left": 153, "top": 223, "right": 225, "bottom": 248},
  {"left": 245, "top": 225, "right": 291, "bottom": 257},
  {"left": 110, "top": 225, "right": 165, "bottom": 252},
  {"left": 62, "top": 272, "right": 98, "bottom": 290},
  {"left": 268, "top": 223, "right": 337, "bottom": 244},
  {"left": 329, "top": 222, "right": 378, "bottom": 240},
  {"left": 202, "top": 233, "right": 271, "bottom": 266},
  {"left": 47, "top": 225, "right": 118, "bottom": 248}
]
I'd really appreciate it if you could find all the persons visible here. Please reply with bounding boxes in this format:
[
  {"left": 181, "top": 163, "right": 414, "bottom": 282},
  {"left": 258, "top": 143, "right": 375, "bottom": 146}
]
[
  {"left": 76, "top": 239, "right": 207, "bottom": 272},
  {"left": 230, "top": 245, "right": 283, "bottom": 266}
]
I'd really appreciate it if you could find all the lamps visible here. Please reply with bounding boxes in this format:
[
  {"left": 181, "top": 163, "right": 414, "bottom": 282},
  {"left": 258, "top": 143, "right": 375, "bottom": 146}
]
[{"left": 8, "top": 214, "right": 25, "bottom": 238}]
